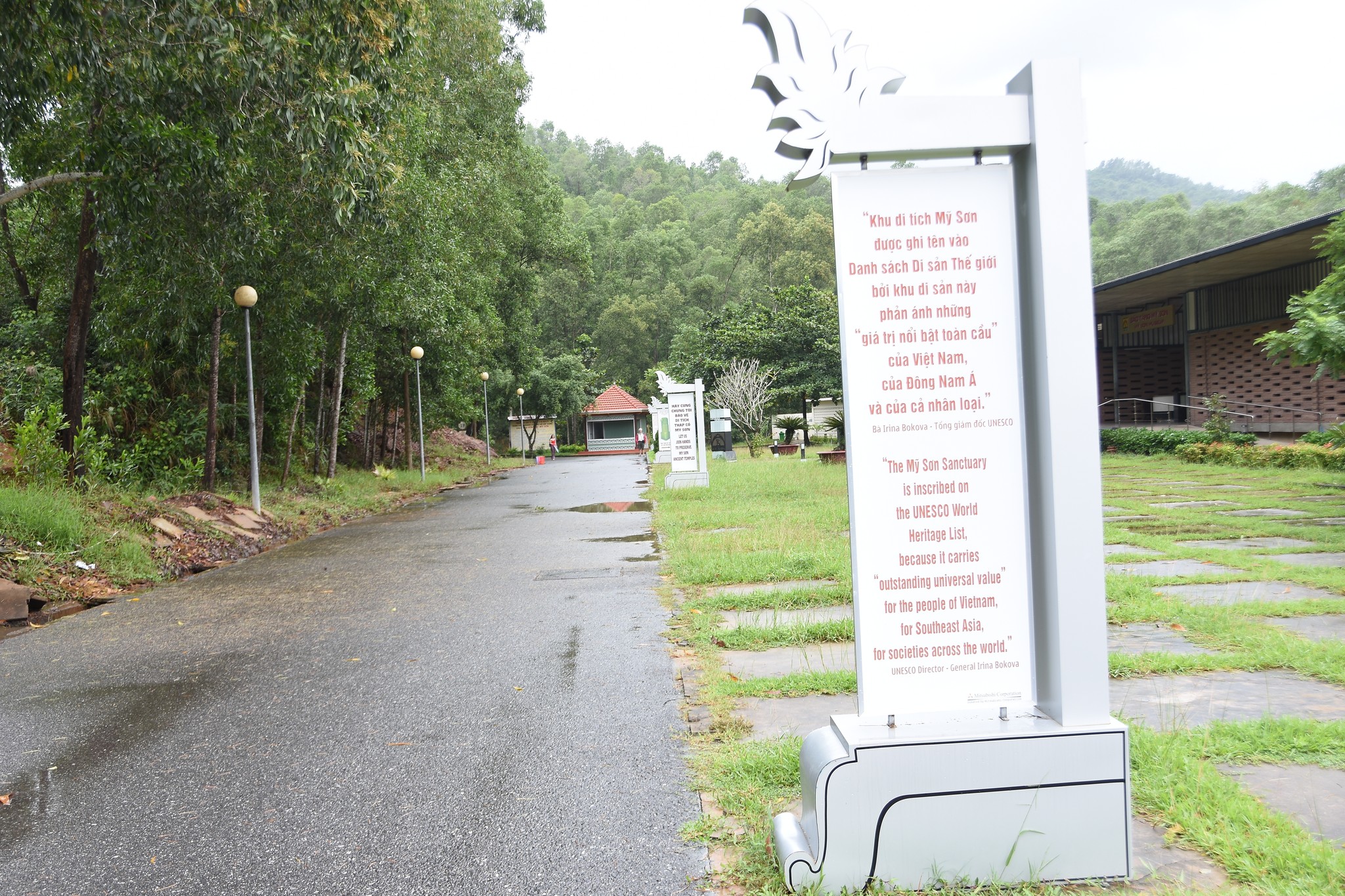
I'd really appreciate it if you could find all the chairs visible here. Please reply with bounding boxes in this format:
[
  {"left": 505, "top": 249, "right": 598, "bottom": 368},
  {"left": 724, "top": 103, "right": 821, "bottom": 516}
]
[{"left": 793, "top": 433, "right": 799, "bottom": 441}]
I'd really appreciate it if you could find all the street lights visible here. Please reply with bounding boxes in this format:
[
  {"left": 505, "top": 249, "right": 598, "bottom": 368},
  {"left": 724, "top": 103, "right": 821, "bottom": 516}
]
[
  {"left": 479, "top": 371, "right": 491, "bottom": 466},
  {"left": 234, "top": 285, "right": 261, "bottom": 514},
  {"left": 410, "top": 346, "right": 426, "bottom": 482},
  {"left": 516, "top": 388, "right": 525, "bottom": 463}
]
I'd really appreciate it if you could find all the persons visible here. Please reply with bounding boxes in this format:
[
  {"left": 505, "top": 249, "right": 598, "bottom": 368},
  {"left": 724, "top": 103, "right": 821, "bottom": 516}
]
[
  {"left": 636, "top": 428, "right": 646, "bottom": 456},
  {"left": 549, "top": 435, "right": 557, "bottom": 460}
]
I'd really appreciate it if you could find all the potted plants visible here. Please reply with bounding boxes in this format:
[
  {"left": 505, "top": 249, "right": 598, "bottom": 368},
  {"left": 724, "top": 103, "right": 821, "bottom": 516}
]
[
  {"left": 768, "top": 413, "right": 812, "bottom": 455},
  {"left": 816, "top": 411, "right": 845, "bottom": 464},
  {"left": 535, "top": 444, "right": 547, "bottom": 465}
]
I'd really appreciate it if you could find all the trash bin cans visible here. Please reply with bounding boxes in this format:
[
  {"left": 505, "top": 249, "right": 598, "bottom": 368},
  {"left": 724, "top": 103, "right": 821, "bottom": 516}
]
[{"left": 779, "top": 432, "right": 784, "bottom": 441}]
